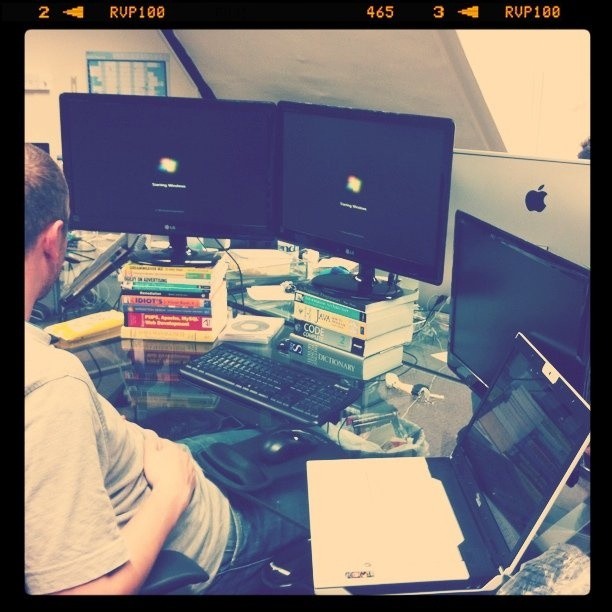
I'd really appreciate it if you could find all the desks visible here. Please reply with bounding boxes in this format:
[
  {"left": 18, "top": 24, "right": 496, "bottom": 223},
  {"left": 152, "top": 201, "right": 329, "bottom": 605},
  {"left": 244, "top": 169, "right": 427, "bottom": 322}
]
[{"left": 30, "top": 274, "right": 591, "bottom": 596}]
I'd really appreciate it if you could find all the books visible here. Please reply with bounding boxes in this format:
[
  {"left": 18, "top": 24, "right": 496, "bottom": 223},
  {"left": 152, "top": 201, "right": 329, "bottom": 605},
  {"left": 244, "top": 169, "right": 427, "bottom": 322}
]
[
  {"left": 120, "top": 260, "right": 228, "bottom": 343},
  {"left": 289, "top": 287, "right": 419, "bottom": 382}
]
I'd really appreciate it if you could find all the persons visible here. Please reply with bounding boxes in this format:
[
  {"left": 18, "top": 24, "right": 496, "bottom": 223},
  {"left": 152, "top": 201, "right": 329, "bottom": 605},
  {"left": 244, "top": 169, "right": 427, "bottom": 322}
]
[{"left": 25, "top": 144, "right": 309, "bottom": 596}]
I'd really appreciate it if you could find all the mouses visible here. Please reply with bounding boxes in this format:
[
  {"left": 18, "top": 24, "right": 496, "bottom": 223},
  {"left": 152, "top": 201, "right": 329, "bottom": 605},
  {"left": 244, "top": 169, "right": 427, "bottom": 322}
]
[{"left": 260, "top": 431, "right": 314, "bottom": 464}]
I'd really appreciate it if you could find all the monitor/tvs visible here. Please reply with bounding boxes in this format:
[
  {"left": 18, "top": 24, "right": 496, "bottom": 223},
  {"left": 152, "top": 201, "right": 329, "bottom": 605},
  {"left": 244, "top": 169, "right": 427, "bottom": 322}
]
[
  {"left": 418, "top": 149, "right": 591, "bottom": 315},
  {"left": 448, "top": 210, "right": 592, "bottom": 488},
  {"left": 59, "top": 93, "right": 276, "bottom": 268},
  {"left": 278, "top": 101, "right": 455, "bottom": 300}
]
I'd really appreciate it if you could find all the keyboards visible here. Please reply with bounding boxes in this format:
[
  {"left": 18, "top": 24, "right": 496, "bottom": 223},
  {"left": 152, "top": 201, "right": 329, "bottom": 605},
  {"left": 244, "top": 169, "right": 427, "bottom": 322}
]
[{"left": 179, "top": 343, "right": 360, "bottom": 425}]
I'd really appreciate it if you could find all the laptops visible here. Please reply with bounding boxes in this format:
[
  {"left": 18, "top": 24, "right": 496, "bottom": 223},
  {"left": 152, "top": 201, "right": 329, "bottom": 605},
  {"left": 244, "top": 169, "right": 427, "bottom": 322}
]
[{"left": 306, "top": 333, "right": 592, "bottom": 597}]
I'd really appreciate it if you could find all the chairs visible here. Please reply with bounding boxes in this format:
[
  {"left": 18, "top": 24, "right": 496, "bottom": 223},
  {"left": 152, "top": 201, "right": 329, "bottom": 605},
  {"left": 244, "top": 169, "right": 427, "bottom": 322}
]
[{"left": 129, "top": 405, "right": 253, "bottom": 594}]
